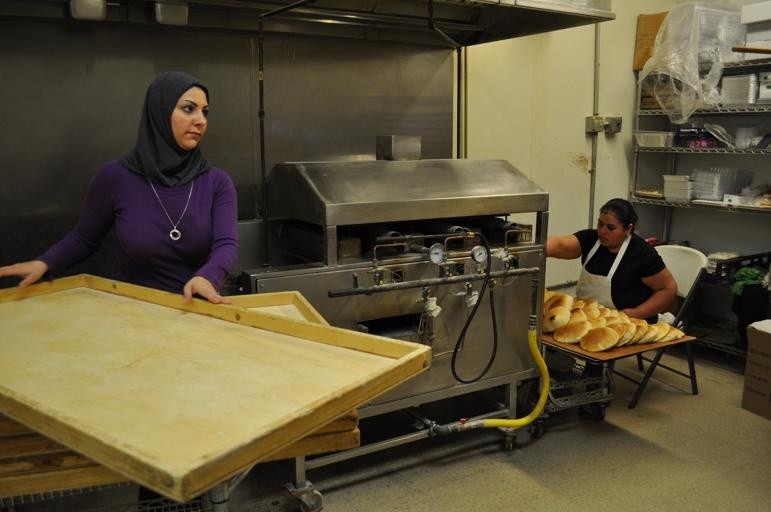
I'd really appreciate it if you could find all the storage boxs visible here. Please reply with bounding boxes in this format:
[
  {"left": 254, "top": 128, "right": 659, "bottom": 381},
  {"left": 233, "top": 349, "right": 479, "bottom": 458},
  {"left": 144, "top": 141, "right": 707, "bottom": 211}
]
[
  {"left": 741, "top": 318, "right": 771, "bottom": 421},
  {"left": 631, "top": 8, "right": 670, "bottom": 71}
]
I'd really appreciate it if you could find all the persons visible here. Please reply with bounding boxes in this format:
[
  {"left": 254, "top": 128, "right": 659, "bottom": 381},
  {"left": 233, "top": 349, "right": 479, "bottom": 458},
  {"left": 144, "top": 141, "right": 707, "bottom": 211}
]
[
  {"left": 539, "top": 199, "right": 679, "bottom": 421},
  {"left": 0, "top": 70, "right": 241, "bottom": 306}
]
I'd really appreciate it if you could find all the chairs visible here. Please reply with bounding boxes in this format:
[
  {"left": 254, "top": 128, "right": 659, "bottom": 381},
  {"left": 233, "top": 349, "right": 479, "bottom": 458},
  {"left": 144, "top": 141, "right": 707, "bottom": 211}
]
[{"left": 606, "top": 244, "right": 711, "bottom": 407}]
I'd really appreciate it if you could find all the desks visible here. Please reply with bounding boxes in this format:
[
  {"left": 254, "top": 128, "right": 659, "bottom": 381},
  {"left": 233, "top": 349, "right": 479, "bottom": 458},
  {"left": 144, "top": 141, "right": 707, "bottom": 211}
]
[{"left": 528, "top": 288, "right": 699, "bottom": 438}]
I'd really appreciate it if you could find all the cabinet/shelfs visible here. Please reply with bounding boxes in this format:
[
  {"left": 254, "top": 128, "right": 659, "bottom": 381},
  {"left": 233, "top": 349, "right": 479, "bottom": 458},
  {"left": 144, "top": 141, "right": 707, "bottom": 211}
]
[{"left": 623, "top": 58, "right": 771, "bottom": 361}]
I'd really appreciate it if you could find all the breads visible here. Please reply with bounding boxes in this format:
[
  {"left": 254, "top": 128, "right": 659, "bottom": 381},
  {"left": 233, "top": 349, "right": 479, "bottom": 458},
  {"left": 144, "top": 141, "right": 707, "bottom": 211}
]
[{"left": 543, "top": 288, "right": 685, "bottom": 352}]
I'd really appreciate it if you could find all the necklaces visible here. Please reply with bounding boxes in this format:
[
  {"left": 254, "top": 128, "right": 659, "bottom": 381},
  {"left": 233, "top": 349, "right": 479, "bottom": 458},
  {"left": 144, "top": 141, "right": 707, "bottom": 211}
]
[{"left": 146, "top": 176, "right": 196, "bottom": 242}]
